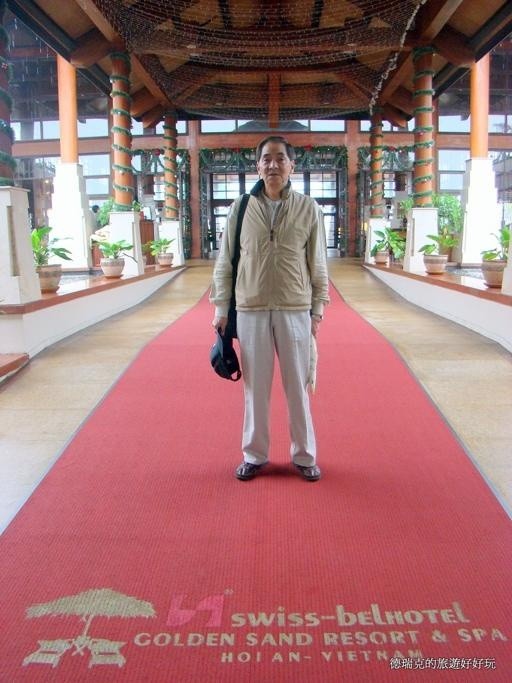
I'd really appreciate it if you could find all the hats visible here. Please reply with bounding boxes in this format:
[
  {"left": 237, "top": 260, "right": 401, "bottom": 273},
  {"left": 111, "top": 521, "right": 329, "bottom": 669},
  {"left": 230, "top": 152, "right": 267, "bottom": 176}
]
[{"left": 210, "top": 329, "right": 241, "bottom": 382}]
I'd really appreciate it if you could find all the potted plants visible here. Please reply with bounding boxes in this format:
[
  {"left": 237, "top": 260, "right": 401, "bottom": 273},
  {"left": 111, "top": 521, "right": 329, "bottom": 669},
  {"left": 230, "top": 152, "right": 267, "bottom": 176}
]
[
  {"left": 368, "top": 226, "right": 510, "bottom": 288},
  {"left": 31, "top": 223, "right": 173, "bottom": 294}
]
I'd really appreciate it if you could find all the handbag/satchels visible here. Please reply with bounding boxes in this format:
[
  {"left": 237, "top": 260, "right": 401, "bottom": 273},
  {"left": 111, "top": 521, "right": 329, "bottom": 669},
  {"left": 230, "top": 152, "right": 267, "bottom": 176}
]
[{"left": 218, "top": 296, "right": 238, "bottom": 338}]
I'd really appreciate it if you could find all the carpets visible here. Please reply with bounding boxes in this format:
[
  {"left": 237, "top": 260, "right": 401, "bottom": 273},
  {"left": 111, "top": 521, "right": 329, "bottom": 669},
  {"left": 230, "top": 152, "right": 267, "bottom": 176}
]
[{"left": 0, "top": 276, "right": 508, "bottom": 682}]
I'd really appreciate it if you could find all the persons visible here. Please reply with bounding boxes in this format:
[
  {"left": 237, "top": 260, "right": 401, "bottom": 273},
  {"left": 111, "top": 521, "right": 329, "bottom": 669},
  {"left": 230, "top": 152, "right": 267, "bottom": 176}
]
[{"left": 209, "top": 133, "right": 331, "bottom": 480}]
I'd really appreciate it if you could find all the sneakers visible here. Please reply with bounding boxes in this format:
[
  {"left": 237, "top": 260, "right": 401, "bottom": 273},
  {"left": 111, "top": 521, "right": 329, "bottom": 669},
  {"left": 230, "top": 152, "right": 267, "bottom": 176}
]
[
  {"left": 294, "top": 461, "right": 321, "bottom": 481},
  {"left": 236, "top": 459, "right": 268, "bottom": 480}
]
